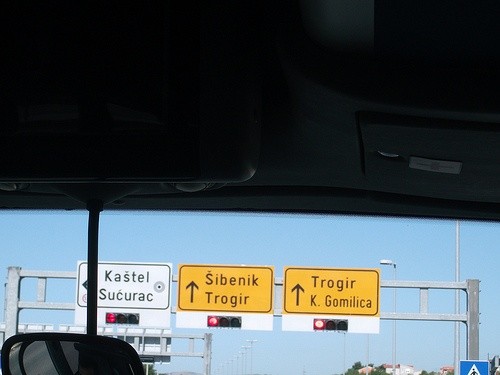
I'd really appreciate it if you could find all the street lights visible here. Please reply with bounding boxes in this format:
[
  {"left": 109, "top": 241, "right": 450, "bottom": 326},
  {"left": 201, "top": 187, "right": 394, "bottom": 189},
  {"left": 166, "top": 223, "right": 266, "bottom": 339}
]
[{"left": 379, "top": 260, "right": 397, "bottom": 375}]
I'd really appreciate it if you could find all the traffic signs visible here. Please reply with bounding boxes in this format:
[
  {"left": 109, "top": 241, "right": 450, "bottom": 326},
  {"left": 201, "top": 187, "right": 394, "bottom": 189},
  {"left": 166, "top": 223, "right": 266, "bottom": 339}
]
[
  {"left": 284, "top": 266, "right": 378, "bottom": 315},
  {"left": 76, "top": 260, "right": 169, "bottom": 308},
  {"left": 178, "top": 264, "right": 272, "bottom": 311}
]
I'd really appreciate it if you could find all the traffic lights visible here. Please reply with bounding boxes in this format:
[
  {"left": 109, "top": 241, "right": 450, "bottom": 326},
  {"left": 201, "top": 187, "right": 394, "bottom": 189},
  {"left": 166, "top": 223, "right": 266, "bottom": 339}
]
[
  {"left": 105, "top": 311, "right": 139, "bottom": 324},
  {"left": 314, "top": 319, "right": 345, "bottom": 330},
  {"left": 207, "top": 315, "right": 241, "bottom": 328}
]
